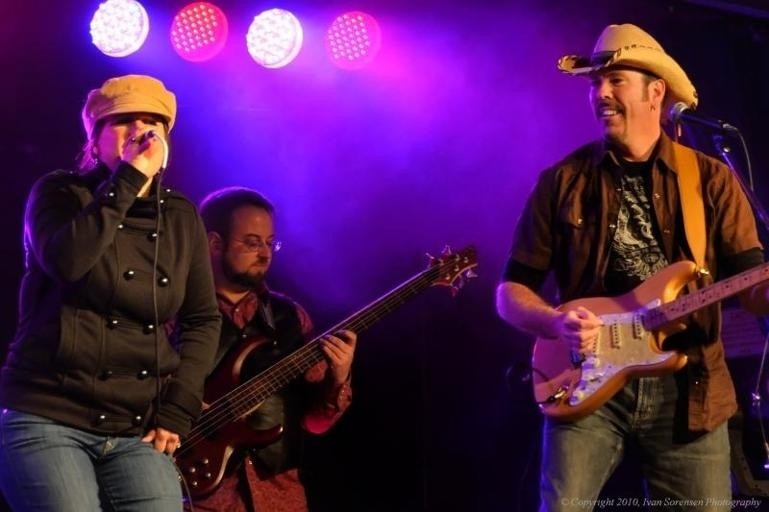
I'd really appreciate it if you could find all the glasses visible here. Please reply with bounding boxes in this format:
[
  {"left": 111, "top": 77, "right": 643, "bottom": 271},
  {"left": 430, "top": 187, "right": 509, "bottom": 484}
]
[{"left": 225, "top": 237, "right": 282, "bottom": 254}]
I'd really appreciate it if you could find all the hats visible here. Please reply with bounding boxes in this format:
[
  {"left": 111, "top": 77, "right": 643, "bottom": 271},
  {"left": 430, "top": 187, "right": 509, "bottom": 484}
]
[
  {"left": 82, "top": 74, "right": 178, "bottom": 142},
  {"left": 555, "top": 23, "right": 700, "bottom": 113}
]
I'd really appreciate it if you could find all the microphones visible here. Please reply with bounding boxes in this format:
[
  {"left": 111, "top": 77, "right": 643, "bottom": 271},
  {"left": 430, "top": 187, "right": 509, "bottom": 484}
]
[
  {"left": 668, "top": 101, "right": 730, "bottom": 131},
  {"left": 138, "top": 129, "right": 155, "bottom": 144}
]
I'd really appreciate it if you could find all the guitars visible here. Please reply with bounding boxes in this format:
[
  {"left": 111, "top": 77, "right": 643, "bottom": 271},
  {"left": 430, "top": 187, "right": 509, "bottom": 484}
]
[
  {"left": 172, "top": 244, "right": 479, "bottom": 500},
  {"left": 531, "top": 259, "right": 768, "bottom": 423}
]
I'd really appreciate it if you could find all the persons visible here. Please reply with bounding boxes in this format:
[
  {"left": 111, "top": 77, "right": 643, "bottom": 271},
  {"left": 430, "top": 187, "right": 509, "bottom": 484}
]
[
  {"left": 0, "top": 74, "right": 223, "bottom": 511},
  {"left": 164, "top": 187, "right": 360, "bottom": 511},
  {"left": 493, "top": 20, "right": 769, "bottom": 512}
]
